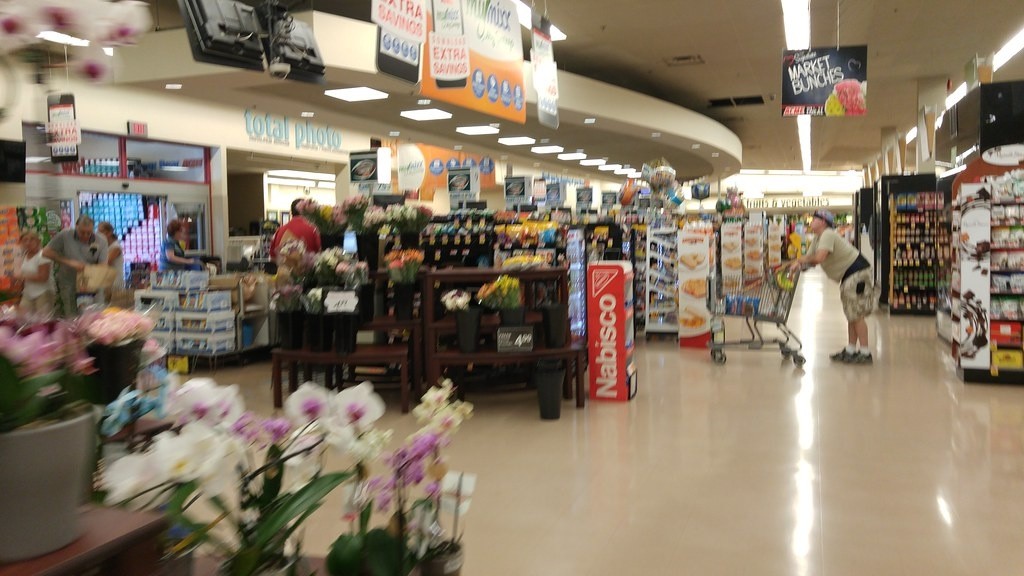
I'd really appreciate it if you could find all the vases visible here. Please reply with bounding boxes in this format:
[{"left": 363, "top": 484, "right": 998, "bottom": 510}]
[
  {"left": 542, "top": 305, "right": 567, "bottom": 346},
  {"left": 335, "top": 308, "right": 360, "bottom": 353},
  {"left": 354, "top": 283, "right": 373, "bottom": 322},
  {"left": 499, "top": 308, "right": 524, "bottom": 327},
  {"left": 399, "top": 231, "right": 420, "bottom": 252},
  {"left": 536, "top": 368, "right": 567, "bottom": 419},
  {"left": 454, "top": 309, "right": 482, "bottom": 353},
  {"left": 303, "top": 313, "right": 333, "bottom": 352},
  {"left": 320, "top": 233, "right": 344, "bottom": 252},
  {"left": 278, "top": 314, "right": 304, "bottom": 351},
  {"left": 0, "top": 403, "right": 100, "bottom": 564},
  {"left": 355, "top": 233, "right": 378, "bottom": 270},
  {"left": 392, "top": 281, "right": 416, "bottom": 319}
]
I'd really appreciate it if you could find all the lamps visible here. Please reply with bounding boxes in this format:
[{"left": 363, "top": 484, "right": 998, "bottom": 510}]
[
  {"left": 324, "top": 86, "right": 642, "bottom": 179},
  {"left": 780, "top": 0, "right": 811, "bottom": 176}
]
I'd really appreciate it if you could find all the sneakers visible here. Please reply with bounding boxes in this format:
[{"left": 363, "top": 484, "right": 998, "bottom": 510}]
[
  {"left": 830, "top": 347, "right": 861, "bottom": 361},
  {"left": 844, "top": 350, "right": 872, "bottom": 364}
]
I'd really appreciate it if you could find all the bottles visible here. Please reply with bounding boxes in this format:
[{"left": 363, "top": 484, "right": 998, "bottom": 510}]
[
  {"left": 893, "top": 210, "right": 936, "bottom": 268},
  {"left": 936, "top": 190, "right": 952, "bottom": 311},
  {"left": 894, "top": 269, "right": 934, "bottom": 311}
]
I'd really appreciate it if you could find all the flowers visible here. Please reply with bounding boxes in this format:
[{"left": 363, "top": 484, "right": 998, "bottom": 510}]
[
  {"left": 92, "top": 366, "right": 477, "bottom": 576},
  {"left": 0, "top": 303, "right": 150, "bottom": 435},
  {"left": 269, "top": 193, "right": 522, "bottom": 314}
]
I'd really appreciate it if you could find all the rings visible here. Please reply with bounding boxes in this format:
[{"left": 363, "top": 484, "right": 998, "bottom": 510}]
[{"left": 787, "top": 273, "right": 789, "bottom": 278}]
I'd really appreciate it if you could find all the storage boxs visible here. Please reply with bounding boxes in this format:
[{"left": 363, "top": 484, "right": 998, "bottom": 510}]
[{"left": 133, "top": 287, "right": 235, "bottom": 356}]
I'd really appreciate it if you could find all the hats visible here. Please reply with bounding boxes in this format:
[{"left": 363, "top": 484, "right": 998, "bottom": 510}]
[{"left": 813, "top": 209, "right": 836, "bottom": 227}]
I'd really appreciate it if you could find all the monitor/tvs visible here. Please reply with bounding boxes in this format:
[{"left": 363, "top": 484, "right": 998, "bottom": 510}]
[{"left": 177, "top": 0, "right": 326, "bottom": 84}]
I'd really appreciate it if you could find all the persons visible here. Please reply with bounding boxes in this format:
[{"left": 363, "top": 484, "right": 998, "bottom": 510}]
[
  {"left": 13, "top": 232, "right": 55, "bottom": 316},
  {"left": 159, "top": 220, "right": 194, "bottom": 274},
  {"left": 779, "top": 209, "right": 874, "bottom": 364},
  {"left": 272, "top": 199, "right": 321, "bottom": 263},
  {"left": 98, "top": 221, "right": 125, "bottom": 291},
  {"left": 44, "top": 217, "right": 107, "bottom": 317}
]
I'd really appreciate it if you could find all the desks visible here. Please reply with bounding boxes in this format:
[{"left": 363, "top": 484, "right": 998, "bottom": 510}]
[{"left": 0, "top": 503, "right": 170, "bottom": 576}]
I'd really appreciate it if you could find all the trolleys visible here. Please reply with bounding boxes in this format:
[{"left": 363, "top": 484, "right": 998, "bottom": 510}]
[{"left": 705, "top": 260, "right": 816, "bottom": 366}]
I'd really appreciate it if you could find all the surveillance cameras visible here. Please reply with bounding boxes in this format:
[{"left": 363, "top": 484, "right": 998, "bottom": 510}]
[{"left": 270, "top": 63, "right": 291, "bottom": 80}]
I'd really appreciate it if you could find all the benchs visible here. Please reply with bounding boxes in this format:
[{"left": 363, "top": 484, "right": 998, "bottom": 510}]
[{"left": 270, "top": 341, "right": 410, "bottom": 415}]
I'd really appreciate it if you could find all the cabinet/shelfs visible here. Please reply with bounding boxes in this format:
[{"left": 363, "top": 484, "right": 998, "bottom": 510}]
[{"left": 441, "top": 80, "right": 1024, "bottom": 387}]
